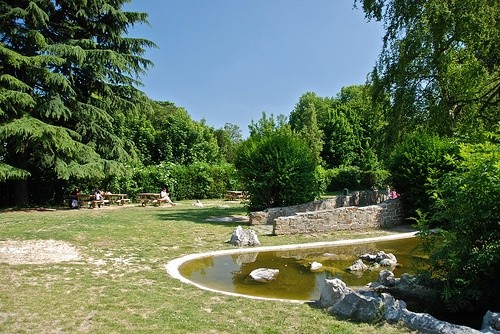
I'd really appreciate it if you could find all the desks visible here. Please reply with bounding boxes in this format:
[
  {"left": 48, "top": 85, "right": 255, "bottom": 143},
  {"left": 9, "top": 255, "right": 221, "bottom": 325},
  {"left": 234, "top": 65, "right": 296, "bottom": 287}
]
[
  {"left": 76, "top": 194, "right": 127, "bottom": 205},
  {"left": 227, "top": 190, "right": 248, "bottom": 200},
  {"left": 136, "top": 193, "right": 161, "bottom": 206}
]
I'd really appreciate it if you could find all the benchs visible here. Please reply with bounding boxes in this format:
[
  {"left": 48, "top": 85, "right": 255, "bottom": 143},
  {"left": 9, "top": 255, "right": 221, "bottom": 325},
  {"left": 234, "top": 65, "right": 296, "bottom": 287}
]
[
  {"left": 225, "top": 194, "right": 250, "bottom": 200},
  {"left": 77, "top": 199, "right": 130, "bottom": 210},
  {"left": 133, "top": 197, "right": 166, "bottom": 207}
]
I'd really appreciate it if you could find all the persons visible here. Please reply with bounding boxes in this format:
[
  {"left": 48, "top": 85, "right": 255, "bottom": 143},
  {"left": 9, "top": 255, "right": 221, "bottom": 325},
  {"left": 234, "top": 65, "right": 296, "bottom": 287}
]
[
  {"left": 68, "top": 186, "right": 105, "bottom": 208},
  {"left": 161, "top": 188, "right": 176, "bottom": 206},
  {"left": 194, "top": 200, "right": 204, "bottom": 207},
  {"left": 389, "top": 188, "right": 399, "bottom": 199}
]
